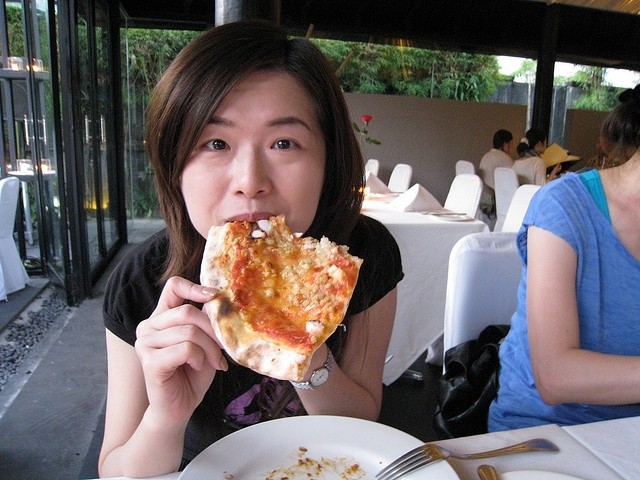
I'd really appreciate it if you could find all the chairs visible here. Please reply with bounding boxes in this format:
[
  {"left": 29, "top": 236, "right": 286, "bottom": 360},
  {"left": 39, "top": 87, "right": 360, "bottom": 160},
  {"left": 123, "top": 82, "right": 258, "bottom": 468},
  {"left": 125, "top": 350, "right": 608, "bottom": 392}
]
[
  {"left": 441, "top": 172, "right": 484, "bottom": 220},
  {"left": 493, "top": 167, "right": 520, "bottom": 232},
  {"left": 361, "top": 158, "right": 379, "bottom": 188},
  {"left": 454, "top": 160, "right": 476, "bottom": 176},
  {"left": 387, "top": 162, "right": 414, "bottom": 196},
  {"left": 442, "top": 231, "right": 527, "bottom": 433},
  {"left": 2, "top": 175, "right": 31, "bottom": 301},
  {"left": 501, "top": 182, "right": 544, "bottom": 233}
]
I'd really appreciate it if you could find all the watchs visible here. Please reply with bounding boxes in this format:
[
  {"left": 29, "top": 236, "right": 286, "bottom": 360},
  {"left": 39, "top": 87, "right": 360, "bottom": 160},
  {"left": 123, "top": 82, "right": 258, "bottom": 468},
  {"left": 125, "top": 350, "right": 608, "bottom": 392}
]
[{"left": 288, "top": 347, "right": 334, "bottom": 390}]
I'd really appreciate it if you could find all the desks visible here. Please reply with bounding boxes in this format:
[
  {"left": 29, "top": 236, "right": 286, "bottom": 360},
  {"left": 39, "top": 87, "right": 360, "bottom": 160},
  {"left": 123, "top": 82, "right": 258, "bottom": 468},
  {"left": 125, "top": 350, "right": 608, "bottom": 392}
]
[{"left": 6, "top": 169, "right": 58, "bottom": 247}]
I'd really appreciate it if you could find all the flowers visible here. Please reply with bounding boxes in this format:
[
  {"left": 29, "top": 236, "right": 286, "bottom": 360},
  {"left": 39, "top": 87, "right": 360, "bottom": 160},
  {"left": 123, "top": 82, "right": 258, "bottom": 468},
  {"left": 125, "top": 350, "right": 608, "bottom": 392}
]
[{"left": 352, "top": 115, "right": 381, "bottom": 164}]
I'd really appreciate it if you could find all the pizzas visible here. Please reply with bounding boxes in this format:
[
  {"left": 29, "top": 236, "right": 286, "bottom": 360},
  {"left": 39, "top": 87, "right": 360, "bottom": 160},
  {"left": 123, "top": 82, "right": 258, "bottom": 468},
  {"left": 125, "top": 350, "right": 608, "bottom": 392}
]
[{"left": 200, "top": 213, "right": 365, "bottom": 382}]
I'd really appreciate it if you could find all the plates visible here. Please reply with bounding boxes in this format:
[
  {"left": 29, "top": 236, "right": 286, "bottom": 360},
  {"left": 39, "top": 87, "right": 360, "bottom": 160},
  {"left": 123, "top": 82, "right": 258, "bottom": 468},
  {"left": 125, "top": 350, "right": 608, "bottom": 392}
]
[
  {"left": 177, "top": 415, "right": 461, "bottom": 480},
  {"left": 440, "top": 214, "right": 473, "bottom": 222}
]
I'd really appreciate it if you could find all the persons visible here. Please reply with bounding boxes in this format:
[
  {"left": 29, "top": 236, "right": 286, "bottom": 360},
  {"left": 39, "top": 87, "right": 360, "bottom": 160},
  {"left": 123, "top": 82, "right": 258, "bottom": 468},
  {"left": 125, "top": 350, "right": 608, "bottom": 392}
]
[
  {"left": 479, "top": 128, "right": 515, "bottom": 189},
  {"left": 513, "top": 127, "right": 562, "bottom": 187},
  {"left": 97, "top": 20, "right": 405, "bottom": 480},
  {"left": 583, "top": 137, "right": 611, "bottom": 169},
  {"left": 487, "top": 84, "right": 640, "bottom": 431}
]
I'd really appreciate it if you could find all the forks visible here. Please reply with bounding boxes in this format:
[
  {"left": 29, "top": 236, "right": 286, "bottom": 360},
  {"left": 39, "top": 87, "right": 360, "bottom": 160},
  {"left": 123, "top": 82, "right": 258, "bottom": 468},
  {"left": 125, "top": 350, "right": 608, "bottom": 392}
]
[{"left": 375, "top": 436, "right": 562, "bottom": 480}]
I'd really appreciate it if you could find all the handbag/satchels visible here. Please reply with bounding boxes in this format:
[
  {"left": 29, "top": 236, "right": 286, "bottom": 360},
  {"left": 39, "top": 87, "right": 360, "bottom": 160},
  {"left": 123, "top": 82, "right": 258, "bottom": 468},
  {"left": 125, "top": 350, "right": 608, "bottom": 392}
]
[{"left": 423, "top": 322, "right": 513, "bottom": 440}]
